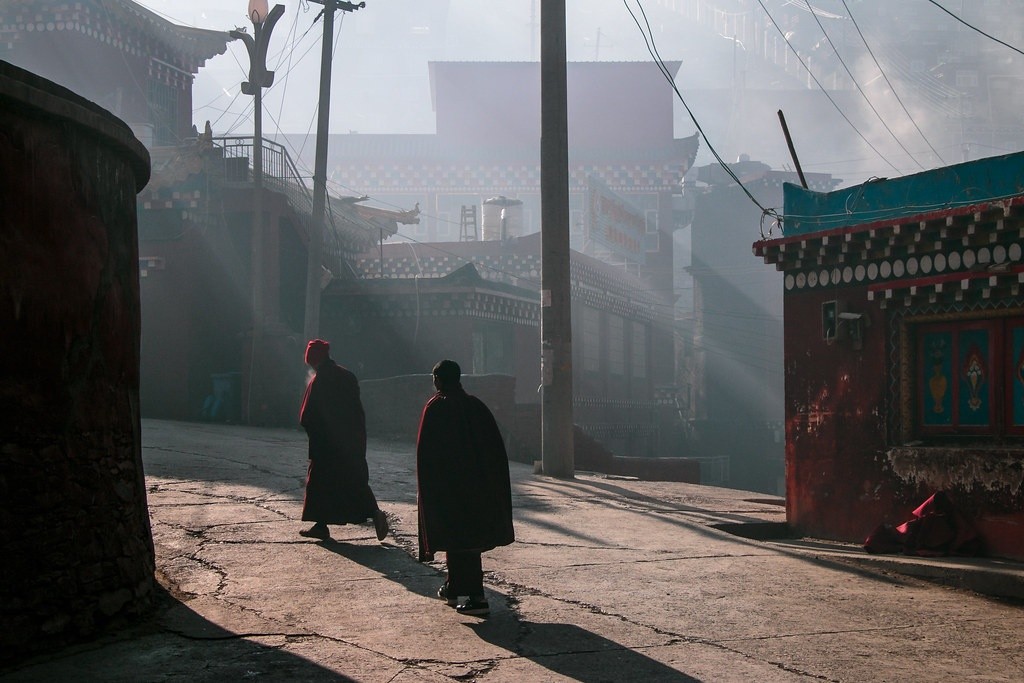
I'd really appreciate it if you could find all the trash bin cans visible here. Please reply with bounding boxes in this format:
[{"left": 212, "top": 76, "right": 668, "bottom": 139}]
[{"left": 209, "top": 371, "right": 241, "bottom": 421}]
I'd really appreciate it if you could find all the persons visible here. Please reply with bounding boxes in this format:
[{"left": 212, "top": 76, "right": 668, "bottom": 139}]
[
  {"left": 299, "top": 339, "right": 389, "bottom": 542},
  {"left": 417, "top": 360, "right": 515, "bottom": 615}
]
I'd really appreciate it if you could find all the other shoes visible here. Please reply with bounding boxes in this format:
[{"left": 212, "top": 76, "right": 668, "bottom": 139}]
[
  {"left": 300, "top": 526, "right": 329, "bottom": 539},
  {"left": 456, "top": 598, "right": 491, "bottom": 615},
  {"left": 437, "top": 584, "right": 456, "bottom": 605},
  {"left": 376, "top": 511, "right": 388, "bottom": 541}
]
